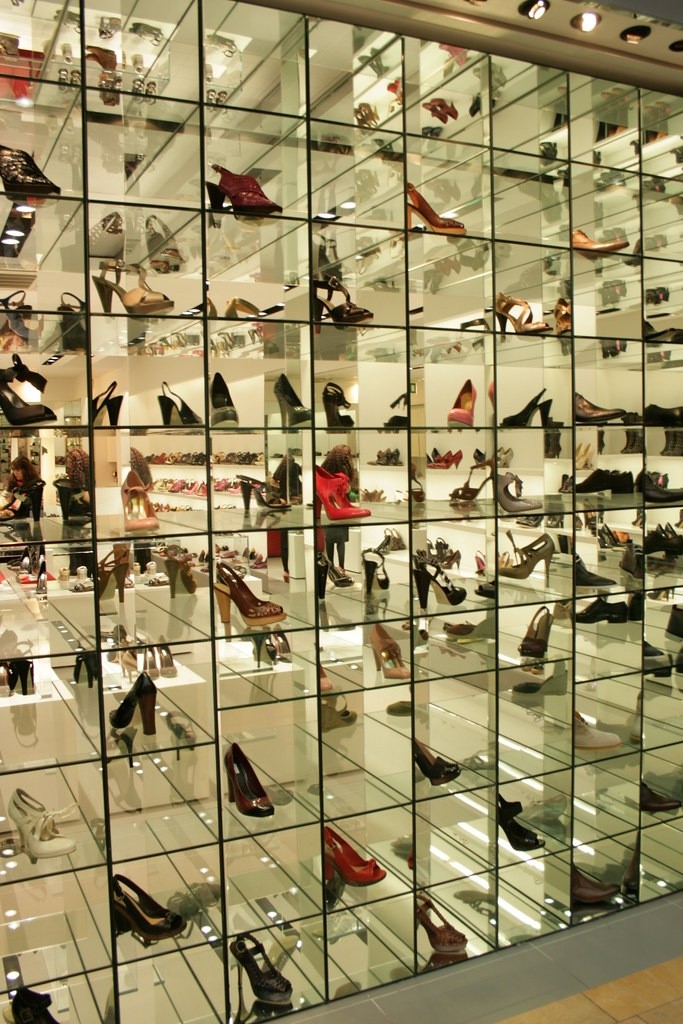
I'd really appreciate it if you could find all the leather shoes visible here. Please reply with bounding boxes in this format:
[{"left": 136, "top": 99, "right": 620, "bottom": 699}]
[
  {"left": 572, "top": 862, "right": 621, "bottom": 903},
  {"left": 641, "top": 781, "right": 681, "bottom": 811},
  {"left": 622, "top": 832, "right": 640, "bottom": 889},
  {"left": 576, "top": 597, "right": 628, "bottom": 623}
]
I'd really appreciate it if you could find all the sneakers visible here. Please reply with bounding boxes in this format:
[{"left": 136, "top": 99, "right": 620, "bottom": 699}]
[
  {"left": 525, "top": 794, "right": 567, "bottom": 825},
  {"left": 576, "top": 553, "right": 620, "bottom": 592}
]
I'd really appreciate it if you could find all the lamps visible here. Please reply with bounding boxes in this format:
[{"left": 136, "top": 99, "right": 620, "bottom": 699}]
[
  {"left": 570, "top": 11, "right": 604, "bottom": 33},
  {"left": 522, "top": 0, "right": 547, "bottom": 21}
]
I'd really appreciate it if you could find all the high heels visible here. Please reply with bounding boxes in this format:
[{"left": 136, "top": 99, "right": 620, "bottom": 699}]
[
  {"left": 415, "top": 738, "right": 462, "bottom": 786},
  {"left": 8, "top": 788, "right": 79, "bottom": 859},
  {"left": 12, "top": 987, "right": 61, "bottom": 1024},
  {"left": 224, "top": 742, "right": 275, "bottom": 817},
  {"left": 416, "top": 894, "right": 467, "bottom": 952},
  {"left": 497, "top": 794, "right": 545, "bottom": 850},
  {"left": 324, "top": 825, "right": 387, "bottom": 887},
  {"left": 112, "top": 875, "right": 187, "bottom": 941},
  {"left": 230, "top": 933, "right": 292, "bottom": 1004},
  {"left": 0, "top": 0, "right": 683, "bottom": 696},
  {"left": 109, "top": 672, "right": 158, "bottom": 735}
]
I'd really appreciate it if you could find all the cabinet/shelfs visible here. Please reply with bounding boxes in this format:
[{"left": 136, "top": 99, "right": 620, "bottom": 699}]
[{"left": 0, "top": 0, "right": 683, "bottom": 1024}]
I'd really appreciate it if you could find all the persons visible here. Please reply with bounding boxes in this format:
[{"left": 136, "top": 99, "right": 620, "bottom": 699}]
[
  {"left": 128, "top": 447, "right": 154, "bottom": 574},
  {"left": 272, "top": 453, "right": 302, "bottom": 584},
  {"left": 65, "top": 449, "right": 92, "bottom": 578},
  {"left": 6, "top": 455, "right": 46, "bottom": 579},
  {"left": 320, "top": 444, "right": 354, "bottom": 576}
]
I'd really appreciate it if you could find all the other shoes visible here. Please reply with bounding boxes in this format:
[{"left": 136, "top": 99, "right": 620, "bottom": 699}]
[
  {"left": 321, "top": 694, "right": 357, "bottom": 733},
  {"left": 574, "top": 711, "right": 621, "bottom": 748},
  {"left": 631, "top": 691, "right": 645, "bottom": 740}
]
